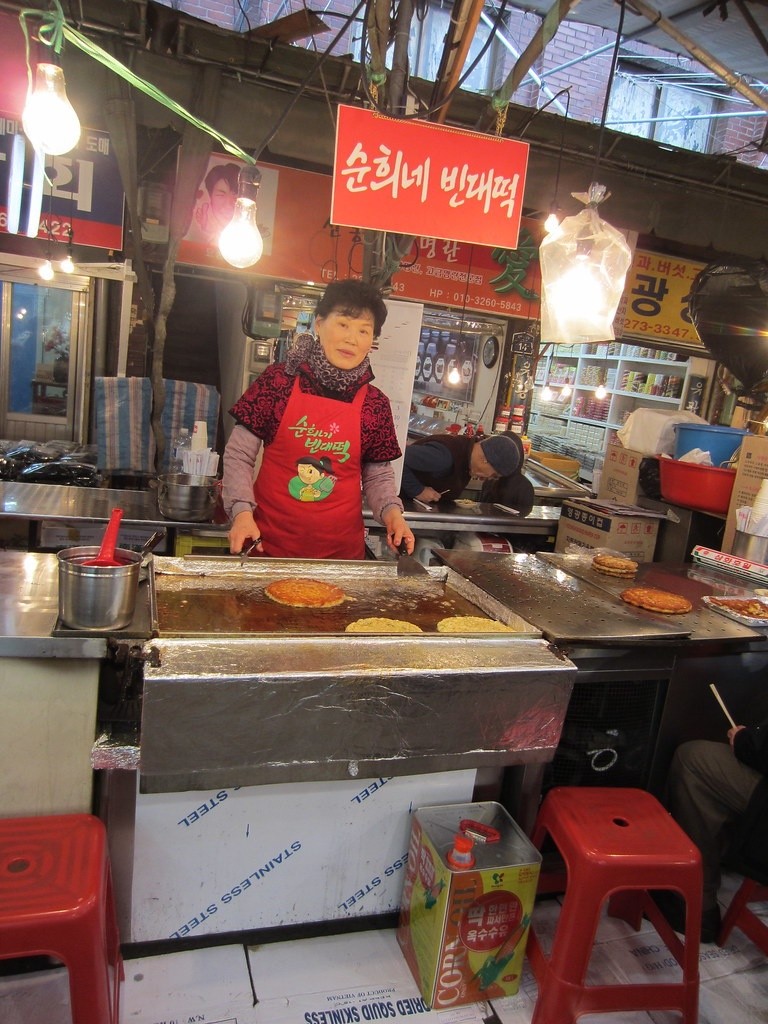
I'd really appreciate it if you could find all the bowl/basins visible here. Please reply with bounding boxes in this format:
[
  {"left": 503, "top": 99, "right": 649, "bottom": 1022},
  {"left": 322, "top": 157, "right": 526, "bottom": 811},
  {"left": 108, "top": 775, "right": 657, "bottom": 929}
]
[
  {"left": 654, "top": 452, "right": 737, "bottom": 514},
  {"left": 455, "top": 499, "right": 478, "bottom": 509},
  {"left": 56, "top": 544, "right": 144, "bottom": 630}
]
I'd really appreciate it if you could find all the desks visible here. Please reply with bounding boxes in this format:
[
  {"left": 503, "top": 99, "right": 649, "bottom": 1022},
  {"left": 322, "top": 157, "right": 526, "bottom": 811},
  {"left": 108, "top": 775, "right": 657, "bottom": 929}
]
[
  {"left": 1, "top": 480, "right": 563, "bottom": 552},
  {"left": 31, "top": 378, "right": 67, "bottom": 417}
]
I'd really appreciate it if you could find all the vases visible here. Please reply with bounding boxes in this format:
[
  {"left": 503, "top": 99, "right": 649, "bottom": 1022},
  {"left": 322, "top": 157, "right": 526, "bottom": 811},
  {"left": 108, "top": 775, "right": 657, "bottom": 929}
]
[{"left": 53, "top": 358, "right": 69, "bottom": 383}]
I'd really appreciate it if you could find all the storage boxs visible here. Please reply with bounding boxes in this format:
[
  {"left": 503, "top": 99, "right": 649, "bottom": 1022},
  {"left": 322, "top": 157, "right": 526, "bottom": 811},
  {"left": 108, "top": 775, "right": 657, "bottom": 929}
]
[
  {"left": 722, "top": 436, "right": 768, "bottom": 556},
  {"left": 554, "top": 499, "right": 661, "bottom": 563},
  {"left": 528, "top": 450, "right": 579, "bottom": 484},
  {"left": 38, "top": 519, "right": 168, "bottom": 552},
  {"left": 599, "top": 445, "right": 646, "bottom": 505}
]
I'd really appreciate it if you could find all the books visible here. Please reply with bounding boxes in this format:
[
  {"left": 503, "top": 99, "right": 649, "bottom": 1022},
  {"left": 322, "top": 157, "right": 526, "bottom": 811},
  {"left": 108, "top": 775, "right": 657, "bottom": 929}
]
[{"left": 567, "top": 497, "right": 667, "bottom": 518}]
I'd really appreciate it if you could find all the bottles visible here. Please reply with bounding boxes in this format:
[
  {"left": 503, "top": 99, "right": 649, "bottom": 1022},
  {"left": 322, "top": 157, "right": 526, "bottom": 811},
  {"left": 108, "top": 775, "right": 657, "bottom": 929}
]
[
  {"left": 494, "top": 404, "right": 525, "bottom": 435},
  {"left": 519, "top": 436, "right": 531, "bottom": 460},
  {"left": 168, "top": 428, "right": 192, "bottom": 473}
]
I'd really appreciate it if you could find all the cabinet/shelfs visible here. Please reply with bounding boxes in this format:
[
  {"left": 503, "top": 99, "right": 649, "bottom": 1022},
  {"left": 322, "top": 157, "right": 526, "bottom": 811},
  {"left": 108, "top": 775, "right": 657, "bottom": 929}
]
[{"left": 528, "top": 340, "right": 690, "bottom": 484}]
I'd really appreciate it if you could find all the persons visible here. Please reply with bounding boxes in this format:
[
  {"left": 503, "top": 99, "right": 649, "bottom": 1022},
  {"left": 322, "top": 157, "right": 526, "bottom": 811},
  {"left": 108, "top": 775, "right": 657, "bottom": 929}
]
[
  {"left": 222, "top": 280, "right": 415, "bottom": 555},
  {"left": 643, "top": 719, "right": 768, "bottom": 943},
  {"left": 397, "top": 430, "right": 534, "bottom": 502}
]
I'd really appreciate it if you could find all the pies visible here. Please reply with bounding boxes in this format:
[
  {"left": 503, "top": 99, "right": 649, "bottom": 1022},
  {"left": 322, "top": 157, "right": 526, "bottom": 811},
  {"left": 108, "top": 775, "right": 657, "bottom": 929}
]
[
  {"left": 622, "top": 586, "right": 693, "bottom": 613},
  {"left": 265, "top": 577, "right": 345, "bottom": 608},
  {"left": 437, "top": 616, "right": 518, "bottom": 632},
  {"left": 346, "top": 617, "right": 423, "bottom": 633},
  {"left": 590, "top": 556, "right": 638, "bottom": 578}
]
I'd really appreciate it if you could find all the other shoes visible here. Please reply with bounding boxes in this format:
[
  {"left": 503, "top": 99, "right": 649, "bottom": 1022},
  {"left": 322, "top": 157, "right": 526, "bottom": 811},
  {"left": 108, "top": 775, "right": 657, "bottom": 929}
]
[{"left": 644, "top": 888, "right": 722, "bottom": 944}]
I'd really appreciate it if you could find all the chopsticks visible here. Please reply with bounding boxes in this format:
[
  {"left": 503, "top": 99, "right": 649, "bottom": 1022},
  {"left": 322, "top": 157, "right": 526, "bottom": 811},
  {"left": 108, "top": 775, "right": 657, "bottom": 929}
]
[{"left": 182, "top": 447, "right": 220, "bottom": 475}]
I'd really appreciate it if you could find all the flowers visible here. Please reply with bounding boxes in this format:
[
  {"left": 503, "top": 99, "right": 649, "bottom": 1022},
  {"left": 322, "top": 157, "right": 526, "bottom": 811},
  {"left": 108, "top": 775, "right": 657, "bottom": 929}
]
[{"left": 44, "top": 325, "right": 69, "bottom": 359}]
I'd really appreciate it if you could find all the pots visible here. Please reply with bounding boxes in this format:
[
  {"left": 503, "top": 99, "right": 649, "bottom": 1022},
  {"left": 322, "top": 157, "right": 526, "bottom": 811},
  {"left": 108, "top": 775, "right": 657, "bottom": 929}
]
[{"left": 148, "top": 474, "right": 220, "bottom": 522}]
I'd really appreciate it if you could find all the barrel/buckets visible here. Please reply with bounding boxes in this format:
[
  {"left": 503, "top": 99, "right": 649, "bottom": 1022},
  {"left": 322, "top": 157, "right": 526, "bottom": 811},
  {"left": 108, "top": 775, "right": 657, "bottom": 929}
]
[
  {"left": 395, "top": 801, "right": 542, "bottom": 1010},
  {"left": 671, "top": 423, "right": 754, "bottom": 472}
]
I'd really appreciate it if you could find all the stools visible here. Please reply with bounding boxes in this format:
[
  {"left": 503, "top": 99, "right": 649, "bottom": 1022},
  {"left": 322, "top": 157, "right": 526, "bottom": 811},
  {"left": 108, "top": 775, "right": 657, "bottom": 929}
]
[
  {"left": 526, "top": 785, "right": 704, "bottom": 1022},
  {"left": 0, "top": 812, "right": 126, "bottom": 1024},
  {"left": 716, "top": 879, "right": 768, "bottom": 960}
]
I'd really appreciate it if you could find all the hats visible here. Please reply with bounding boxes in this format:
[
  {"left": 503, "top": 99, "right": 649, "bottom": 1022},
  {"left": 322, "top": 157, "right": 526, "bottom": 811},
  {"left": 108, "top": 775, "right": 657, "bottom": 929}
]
[{"left": 480, "top": 435, "right": 520, "bottom": 478}]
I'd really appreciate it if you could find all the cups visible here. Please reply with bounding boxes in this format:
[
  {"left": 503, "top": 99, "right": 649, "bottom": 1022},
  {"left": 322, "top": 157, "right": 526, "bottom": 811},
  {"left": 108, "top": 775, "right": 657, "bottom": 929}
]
[{"left": 191, "top": 420, "right": 208, "bottom": 451}]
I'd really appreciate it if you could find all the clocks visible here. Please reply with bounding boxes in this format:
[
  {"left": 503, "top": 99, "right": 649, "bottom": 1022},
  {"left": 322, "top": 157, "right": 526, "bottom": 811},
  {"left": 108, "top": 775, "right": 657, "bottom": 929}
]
[{"left": 483, "top": 335, "right": 499, "bottom": 368}]
